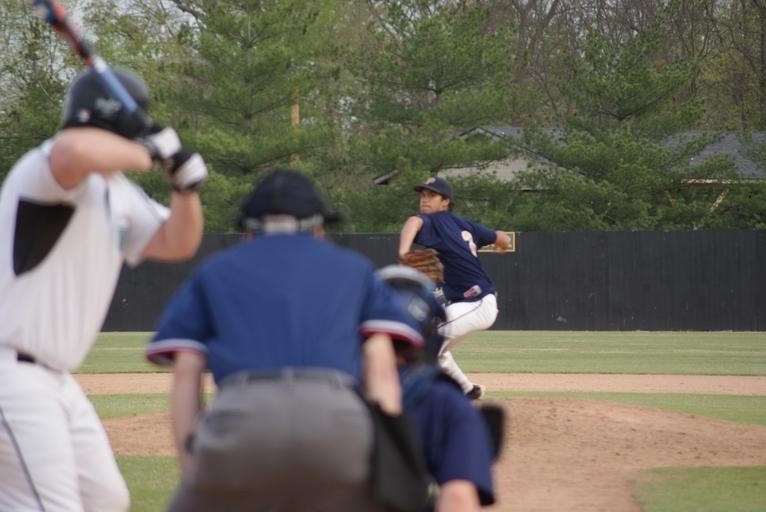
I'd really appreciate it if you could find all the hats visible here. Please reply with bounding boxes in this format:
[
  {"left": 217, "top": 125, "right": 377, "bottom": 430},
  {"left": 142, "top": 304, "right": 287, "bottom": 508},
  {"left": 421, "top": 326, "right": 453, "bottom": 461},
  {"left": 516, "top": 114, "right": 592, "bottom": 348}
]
[
  {"left": 414, "top": 176, "right": 452, "bottom": 196},
  {"left": 234, "top": 170, "right": 343, "bottom": 233}
]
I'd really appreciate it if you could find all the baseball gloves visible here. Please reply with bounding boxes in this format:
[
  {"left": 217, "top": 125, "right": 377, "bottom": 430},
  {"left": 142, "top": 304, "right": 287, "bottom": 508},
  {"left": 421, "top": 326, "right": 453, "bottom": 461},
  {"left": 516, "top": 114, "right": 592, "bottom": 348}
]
[{"left": 399, "top": 249, "right": 444, "bottom": 283}]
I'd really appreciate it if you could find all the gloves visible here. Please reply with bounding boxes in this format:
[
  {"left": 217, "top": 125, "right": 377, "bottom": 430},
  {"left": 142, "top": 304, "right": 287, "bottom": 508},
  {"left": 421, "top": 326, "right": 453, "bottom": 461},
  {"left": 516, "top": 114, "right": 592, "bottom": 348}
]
[{"left": 145, "top": 125, "right": 207, "bottom": 192}]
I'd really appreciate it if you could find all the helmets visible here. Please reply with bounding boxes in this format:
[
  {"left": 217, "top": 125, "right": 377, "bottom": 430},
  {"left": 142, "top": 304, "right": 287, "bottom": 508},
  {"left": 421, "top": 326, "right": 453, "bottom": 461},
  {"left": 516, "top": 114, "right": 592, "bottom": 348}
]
[
  {"left": 374, "top": 264, "right": 446, "bottom": 334},
  {"left": 57, "top": 68, "right": 148, "bottom": 140}
]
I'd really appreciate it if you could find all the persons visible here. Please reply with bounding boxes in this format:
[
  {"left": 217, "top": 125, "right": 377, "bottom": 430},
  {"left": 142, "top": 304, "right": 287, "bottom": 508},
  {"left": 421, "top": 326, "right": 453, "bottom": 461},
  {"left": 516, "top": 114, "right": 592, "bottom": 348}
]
[
  {"left": 0, "top": 66, "right": 205, "bottom": 512},
  {"left": 398, "top": 175, "right": 510, "bottom": 399}
]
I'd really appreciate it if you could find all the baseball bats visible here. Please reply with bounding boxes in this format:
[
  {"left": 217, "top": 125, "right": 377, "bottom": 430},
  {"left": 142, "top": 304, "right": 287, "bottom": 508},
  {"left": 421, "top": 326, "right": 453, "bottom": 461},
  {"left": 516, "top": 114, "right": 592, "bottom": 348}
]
[{"left": 35, "top": 1, "right": 200, "bottom": 189}]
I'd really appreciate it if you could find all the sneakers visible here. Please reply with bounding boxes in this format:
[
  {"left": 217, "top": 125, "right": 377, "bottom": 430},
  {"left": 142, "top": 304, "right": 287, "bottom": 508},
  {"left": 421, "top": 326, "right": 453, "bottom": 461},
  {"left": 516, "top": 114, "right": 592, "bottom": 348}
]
[{"left": 465, "top": 385, "right": 482, "bottom": 399}]
